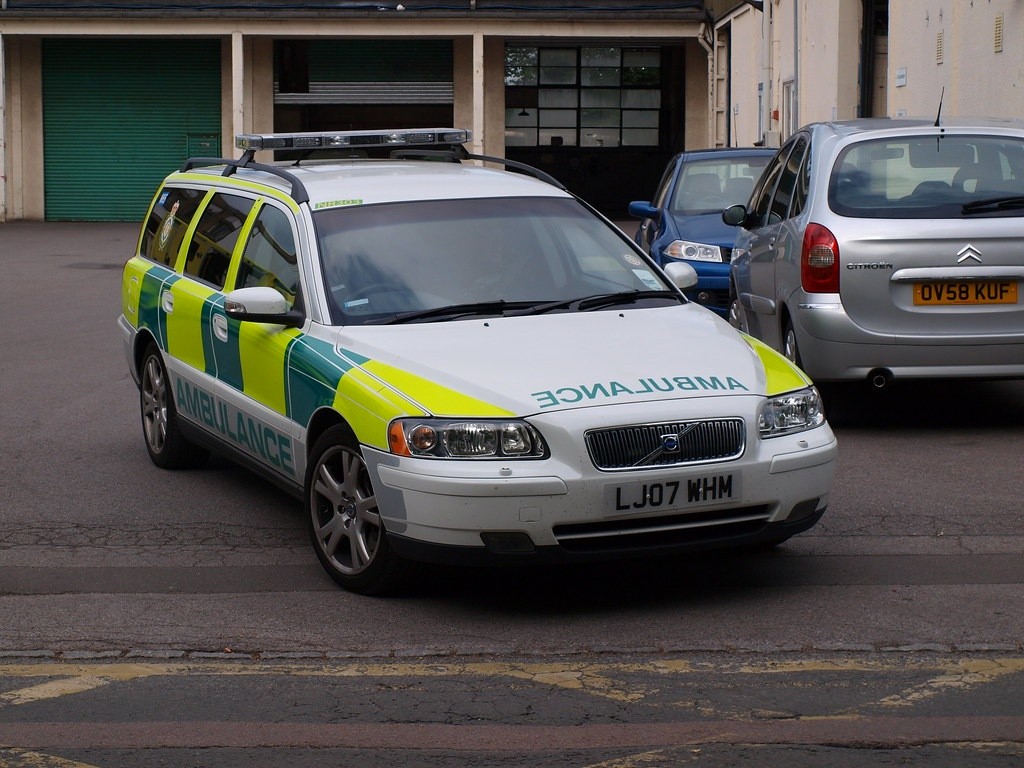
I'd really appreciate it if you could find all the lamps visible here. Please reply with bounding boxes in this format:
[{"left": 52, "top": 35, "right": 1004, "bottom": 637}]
[{"left": 518, "top": 108, "right": 529, "bottom": 116}]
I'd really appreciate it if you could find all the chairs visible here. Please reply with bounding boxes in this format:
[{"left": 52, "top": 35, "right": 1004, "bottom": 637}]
[{"left": 688, "top": 174, "right": 754, "bottom": 203}]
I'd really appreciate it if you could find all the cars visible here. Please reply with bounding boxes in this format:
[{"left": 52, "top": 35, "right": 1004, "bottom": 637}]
[
  {"left": 723, "top": 118, "right": 1024, "bottom": 425},
  {"left": 630, "top": 147, "right": 872, "bottom": 320}
]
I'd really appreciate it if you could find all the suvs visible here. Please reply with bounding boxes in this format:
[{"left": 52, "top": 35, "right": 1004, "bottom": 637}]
[{"left": 118, "top": 128, "right": 838, "bottom": 595}]
